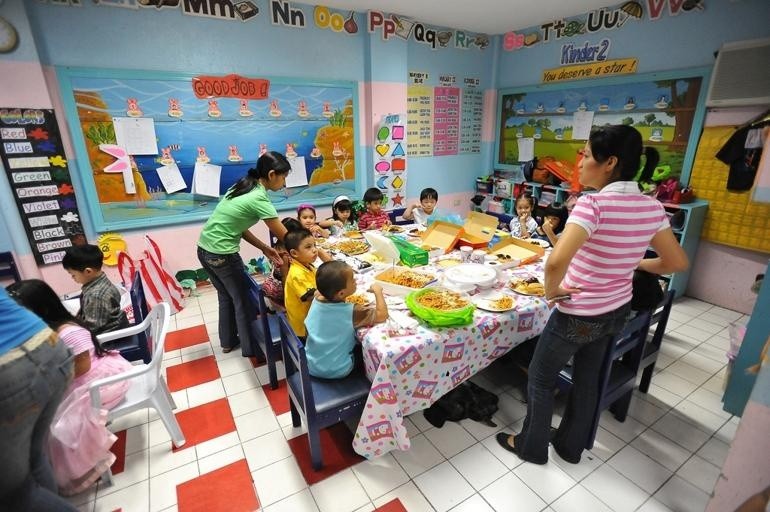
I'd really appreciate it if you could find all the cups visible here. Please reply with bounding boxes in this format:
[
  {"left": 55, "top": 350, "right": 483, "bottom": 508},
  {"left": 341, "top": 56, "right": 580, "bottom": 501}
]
[
  {"left": 460, "top": 245, "right": 473, "bottom": 264},
  {"left": 473, "top": 250, "right": 487, "bottom": 264}
]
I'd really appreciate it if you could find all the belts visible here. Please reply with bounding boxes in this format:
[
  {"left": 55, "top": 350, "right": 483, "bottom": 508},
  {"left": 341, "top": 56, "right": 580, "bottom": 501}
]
[{"left": 0, "top": 327, "right": 52, "bottom": 366}]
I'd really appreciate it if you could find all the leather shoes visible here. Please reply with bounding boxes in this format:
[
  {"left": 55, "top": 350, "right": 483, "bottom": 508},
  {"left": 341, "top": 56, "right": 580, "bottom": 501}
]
[
  {"left": 549, "top": 426, "right": 559, "bottom": 442},
  {"left": 497, "top": 432, "right": 517, "bottom": 450}
]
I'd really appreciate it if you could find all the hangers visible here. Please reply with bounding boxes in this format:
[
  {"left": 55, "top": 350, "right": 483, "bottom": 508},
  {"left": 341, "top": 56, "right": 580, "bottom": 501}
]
[{"left": 751, "top": 117, "right": 770, "bottom": 128}]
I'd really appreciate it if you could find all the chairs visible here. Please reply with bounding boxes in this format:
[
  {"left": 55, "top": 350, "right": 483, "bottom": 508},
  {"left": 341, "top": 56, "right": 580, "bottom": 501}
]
[
  {"left": 90, "top": 301, "right": 186, "bottom": 485},
  {"left": 95, "top": 271, "right": 151, "bottom": 368},
  {"left": 269, "top": 222, "right": 281, "bottom": 248},
  {"left": 554, "top": 304, "right": 651, "bottom": 448},
  {"left": 278, "top": 312, "right": 371, "bottom": 472},
  {"left": 609, "top": 290, "right": 676, "bottom": 417},
  {"left": 242, "top": 269, "right": 289, "bottom": 390},
  {"left": 387, "top": 208, "right": 415, "bottom": 224}
]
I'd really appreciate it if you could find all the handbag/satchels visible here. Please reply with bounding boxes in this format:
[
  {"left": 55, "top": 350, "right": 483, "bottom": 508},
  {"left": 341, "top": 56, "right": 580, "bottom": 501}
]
[{"left": 523, "top": 156, "right": 575, "bottom": 184}]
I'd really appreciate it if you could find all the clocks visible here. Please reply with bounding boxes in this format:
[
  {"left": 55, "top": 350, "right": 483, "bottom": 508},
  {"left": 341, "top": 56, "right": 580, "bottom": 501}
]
[{"left": 1, "top": 16, "right": 18, "bottom": 53}]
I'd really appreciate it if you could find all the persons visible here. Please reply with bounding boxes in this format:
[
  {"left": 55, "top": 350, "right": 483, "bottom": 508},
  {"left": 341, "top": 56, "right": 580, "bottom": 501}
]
[
  {"left": 1, "top": 282, "right": 80, "bottom": 512},
  {"left": 304, "top": 261, "right": 387, "bottom": 381},
  {"left": 359, "top": 188, "right": 391, "bottom": 229},
  {"left": 297, "top": 204, "right": 328, "bottom": 239},
  {"left": 564, "top": 252, "right": 664, "bottom": 367},
  {"left": 262, "top": 218, "right": 300, "bottom": 314},
  {"left": 287, "top": 229, "right": 333, "bottom": 343},
  {"left": 496, "top": 125, "right": 690, "bottom": 465},
  {"left": 532, "top": 202, "right": 570, "bottom": 247},
  {"left": 319, "top": 195, "right": 358, "bottom": 235},
  {"left": 61, "top": 247, "right": 129, "bottom": 344},
  {"left": 502, "top": 192, "right": 537, "bottom": 237},
  {"left": 198, "top": 152, "right": 290, "bottom": 357},
  {"left": 403, "top": 189, "right": 438, "bottom": 222},
  {"left": 5, "top": 281, "right": 134, "bottom": 498}
]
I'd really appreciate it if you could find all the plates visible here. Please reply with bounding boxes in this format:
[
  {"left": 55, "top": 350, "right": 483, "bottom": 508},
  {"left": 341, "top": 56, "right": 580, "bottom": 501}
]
[
  {"left": 407, "top": 226, "right": 428, "bottom": 237},
  {"left": 471, "top": 293, "right": 518, "bottom": 312},
  {"left": 508, "top": 276, "right": 546, "bottom": 295},
  {"left": 347, "top": 291, "right": 376, "bottom": 306},
  {"left": 446, "top": 263, "right": 497, "bottom": 289},
  {"left": 378, "top": 225, "right": 405, "bottom": 234},
  {"left": 521, "top": 238, "right": 550, "bottom": 248},
  {"left": 314, "top": 238, "right": 327, "bottom": 248}
]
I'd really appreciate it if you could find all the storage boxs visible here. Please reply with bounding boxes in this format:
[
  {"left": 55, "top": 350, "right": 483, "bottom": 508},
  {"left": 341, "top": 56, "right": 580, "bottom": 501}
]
[{"left": 477, "top": 179, "right": 557, "bottom": 226}]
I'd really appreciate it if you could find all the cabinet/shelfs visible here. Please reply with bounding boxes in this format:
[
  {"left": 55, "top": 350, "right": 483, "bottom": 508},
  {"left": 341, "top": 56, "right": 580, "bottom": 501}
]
[
  {"left": 722, "top": 263, "right": 770, "bottom": 418},
  {"left": 474, "top": 175, "right": 710, "bottom": 301}
]
